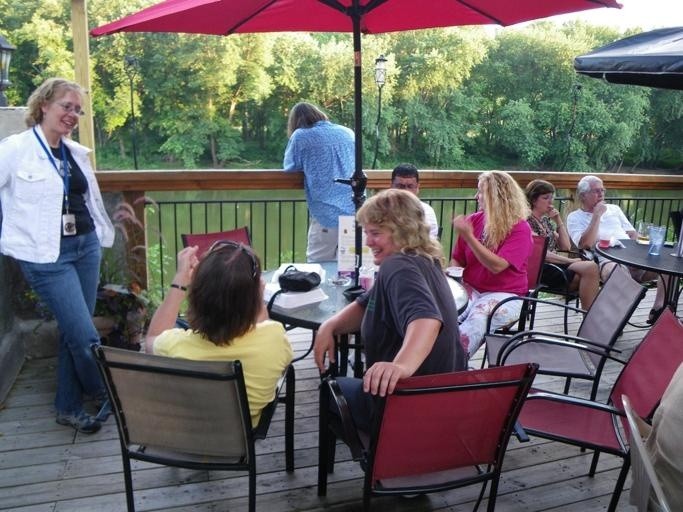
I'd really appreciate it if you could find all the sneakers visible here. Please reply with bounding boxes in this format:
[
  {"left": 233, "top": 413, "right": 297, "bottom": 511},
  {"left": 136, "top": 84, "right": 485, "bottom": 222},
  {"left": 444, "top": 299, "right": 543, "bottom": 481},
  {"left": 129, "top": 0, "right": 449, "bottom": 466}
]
[
  {"left": 56, "top": 409, "right": 100, "bottom": 433},
  {"left": 96, "top": 398, "right": 115, "bottom": 415}
]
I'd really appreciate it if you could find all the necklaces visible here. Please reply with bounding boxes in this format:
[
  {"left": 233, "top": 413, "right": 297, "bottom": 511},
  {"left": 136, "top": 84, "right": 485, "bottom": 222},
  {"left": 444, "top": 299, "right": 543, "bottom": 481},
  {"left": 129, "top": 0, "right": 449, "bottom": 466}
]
[{"left": 531, "top": 213, "right": 549, "bottom": 235}]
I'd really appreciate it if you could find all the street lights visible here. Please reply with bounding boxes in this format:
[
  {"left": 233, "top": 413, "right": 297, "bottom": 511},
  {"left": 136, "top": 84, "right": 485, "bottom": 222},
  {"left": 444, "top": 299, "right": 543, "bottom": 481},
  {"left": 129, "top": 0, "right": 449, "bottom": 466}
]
[
  {"left": 371, "top": 54, "right": 387, "bottom": 171},
  {"left": 559, "top": 81, "right": 584, "bottom": 171},
  {"left": 123, "top": 56, "right": 138, "bottom": 171}
]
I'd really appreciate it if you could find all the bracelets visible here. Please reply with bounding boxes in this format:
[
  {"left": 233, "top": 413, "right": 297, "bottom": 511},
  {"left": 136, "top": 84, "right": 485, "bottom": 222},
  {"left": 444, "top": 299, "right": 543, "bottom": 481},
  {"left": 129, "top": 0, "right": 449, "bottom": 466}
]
[{"left": 170, "top": 284, "right": 188, "bottom": 292}]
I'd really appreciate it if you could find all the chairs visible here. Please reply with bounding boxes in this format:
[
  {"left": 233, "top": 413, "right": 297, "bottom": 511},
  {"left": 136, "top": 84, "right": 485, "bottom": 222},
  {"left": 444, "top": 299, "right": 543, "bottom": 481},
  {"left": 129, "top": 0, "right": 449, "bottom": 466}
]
[
  {"left": 669, "top": 210, "right": 682, "bottom": 242},
  {"left": 482, "top": 262, "right": 648, "bottom": 453},
  {"left": 527, "top": 234, "right": 626, "bottom": 341},
  {"left": 316, "top": 361, "right": 540, "bottom": 512},
  {"left": 494, "top": 235, "right": 551, "bottom": 338},
  {"left": 488, "top": 304, "right": 681, "bottom": 512},
  {"left": 88, "top": 342, "right": 295, "bottom": 512},
  {"left": 180, "top": 225, "right": 254, "bottom": 263}
]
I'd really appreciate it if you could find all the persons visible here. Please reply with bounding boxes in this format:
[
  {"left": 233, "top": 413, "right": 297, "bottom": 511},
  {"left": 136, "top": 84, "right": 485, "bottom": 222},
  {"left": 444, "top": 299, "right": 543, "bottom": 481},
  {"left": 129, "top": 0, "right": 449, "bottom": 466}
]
[
  {"left": 283, "top": 103, "right": 367, "bottom": 264},
  {"left": 0, "top": 79, "right": 115, "bottom": 434},
  {"left": 314, "top": 189, "right": 465, "bottom": 499},
  {"left": 647, "top": 358, "right": 683, "bottom": 512},
  {"left": 565, "top": 175, "right": 677, "bottom": 325},
  {"left": 524, "top": 179, "right": 600, "bottom": 320},
  {"left": 144, "top": 239, "right": 293, "bottom": 442},
  {"left": 449, "top": 171, "right": 533, "bottom": 360},
  {"left": 347, "top": 163, "right": 438, "bottom": 367}
]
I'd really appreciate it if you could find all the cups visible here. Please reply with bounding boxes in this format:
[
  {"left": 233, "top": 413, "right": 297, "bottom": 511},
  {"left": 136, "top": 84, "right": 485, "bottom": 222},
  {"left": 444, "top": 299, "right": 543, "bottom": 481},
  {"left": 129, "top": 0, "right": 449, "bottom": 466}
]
[
  {"left": 647, "top": 225, "right": 666, "bottom": 256},
  {"left": 599, "top": 232, "right": 611, "bottom": 248},
  {"left": 331, "top": 266, "right": 352, "bottom": 287},
  {"left": 634, "top": 219, "right": 654, "bottom": 245},
  {"left": 358, "top": 265, "right": 374, "bottom": 291}
]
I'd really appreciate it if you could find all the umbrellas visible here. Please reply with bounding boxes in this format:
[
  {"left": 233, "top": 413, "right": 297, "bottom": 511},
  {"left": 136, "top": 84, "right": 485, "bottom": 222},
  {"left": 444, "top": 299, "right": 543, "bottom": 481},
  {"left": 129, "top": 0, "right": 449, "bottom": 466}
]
[{"left": 89, "top": 0, "right": 623, "bottom": 286}]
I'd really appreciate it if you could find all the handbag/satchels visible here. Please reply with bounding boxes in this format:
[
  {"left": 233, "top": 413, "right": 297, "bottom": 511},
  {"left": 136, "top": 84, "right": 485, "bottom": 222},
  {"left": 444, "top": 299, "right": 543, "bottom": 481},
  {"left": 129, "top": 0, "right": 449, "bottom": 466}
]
[{"left": 279, "top": 270, "right": 321, "bottom": 291}]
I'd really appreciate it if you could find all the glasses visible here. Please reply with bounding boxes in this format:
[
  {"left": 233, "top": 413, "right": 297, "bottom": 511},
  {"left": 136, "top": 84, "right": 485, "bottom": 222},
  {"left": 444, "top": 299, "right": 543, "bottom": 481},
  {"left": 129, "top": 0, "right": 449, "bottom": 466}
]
[
  {"left": 55, "top": 101, "right": 85, "bottom": 116},
  {"left": 210, "top": 240, "right": 259, "bottom": 278}
]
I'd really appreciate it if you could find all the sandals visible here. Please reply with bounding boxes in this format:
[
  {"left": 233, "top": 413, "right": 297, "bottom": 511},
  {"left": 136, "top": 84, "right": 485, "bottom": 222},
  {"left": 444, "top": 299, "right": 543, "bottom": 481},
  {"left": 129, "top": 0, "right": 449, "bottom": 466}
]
[{"left": 646, "top": 307, "right": 663, "bottom": 324}]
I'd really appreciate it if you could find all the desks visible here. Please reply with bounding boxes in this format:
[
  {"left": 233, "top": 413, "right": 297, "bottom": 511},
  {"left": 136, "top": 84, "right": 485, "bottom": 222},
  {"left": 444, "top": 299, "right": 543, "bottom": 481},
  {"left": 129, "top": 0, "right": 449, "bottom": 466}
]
[
  {"left": 260, "top": 258, "right": 470, "bottom": 376},
  {"left": 596, "top": 238, "right": 683, "bottom": 326}
]
